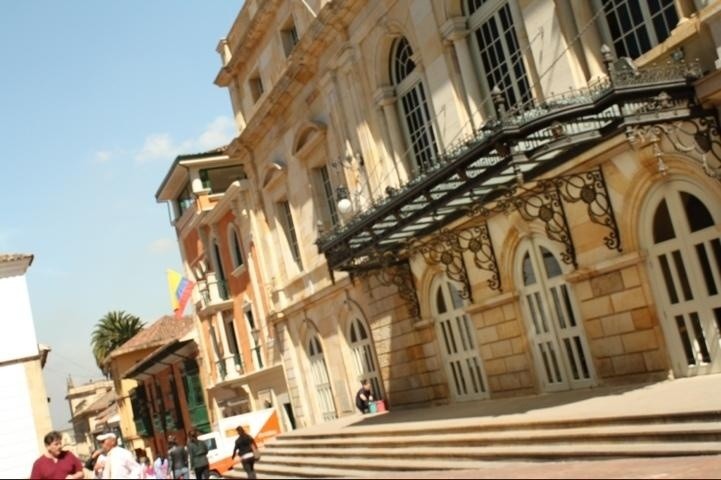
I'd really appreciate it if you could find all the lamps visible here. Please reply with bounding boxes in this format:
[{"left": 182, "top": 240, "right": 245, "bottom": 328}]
[{"left": 330, "top": 151, "right": 364, "bottom": 213}]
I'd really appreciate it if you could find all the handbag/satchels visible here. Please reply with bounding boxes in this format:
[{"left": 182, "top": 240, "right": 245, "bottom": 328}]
[{"left": 254, "top": 450, "right": 260, "bottom": 460}]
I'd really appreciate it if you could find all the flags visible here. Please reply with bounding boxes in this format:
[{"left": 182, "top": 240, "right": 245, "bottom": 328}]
[{"left": 167, "top": 269, "right": 198, "bottom": 320}]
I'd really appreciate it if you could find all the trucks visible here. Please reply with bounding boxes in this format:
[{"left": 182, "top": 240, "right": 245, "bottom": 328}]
[{"left": 196, "top": 409, "right": 282, "bottom": 480}]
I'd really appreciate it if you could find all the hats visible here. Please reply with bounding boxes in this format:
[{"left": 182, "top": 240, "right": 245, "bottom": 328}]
[{"left": 96, "top": 433, "right": 117, "bottom": 441}]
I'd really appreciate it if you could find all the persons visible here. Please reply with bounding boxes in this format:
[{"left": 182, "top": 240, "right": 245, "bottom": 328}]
[
  {"left": 95, "top": 432, "right": 141, "bottom": 479},
  {"left": 88, "top": 444, "right": 107, "bottom": 480},
  {"left": 27, "top": 430, "right": 83, "bottom": 480},
  {"left": 142, "top": 457, "right": 157, "bottom": 479},
  {"left": 153, "top": 453, "right": 170, "bottom": 480},
  {"left": 165, "top": 433, "right": 191, "bottom": 480},
  {"left": 184, "top": 430, "right": 211, "bottom": 480},
  {"left": 355, "top": 380, "right": 374, "bottom": 415},
  {"left": 230, "top": 426, "right": 261, "bottom": 479}
]
